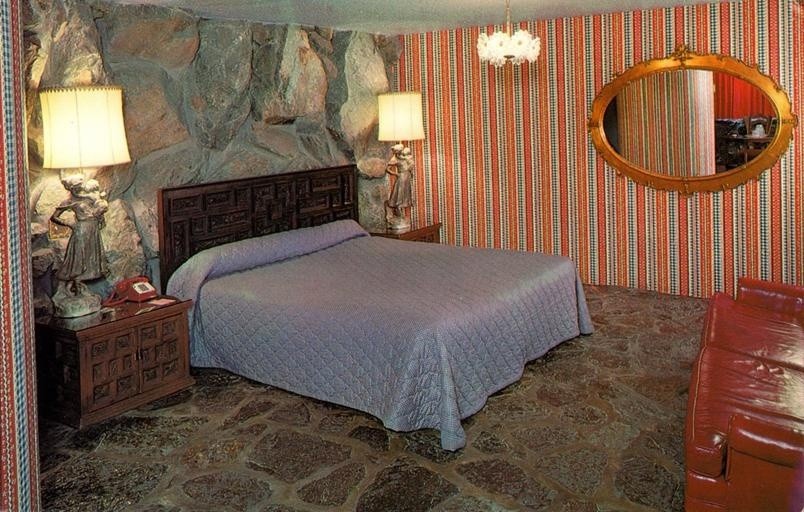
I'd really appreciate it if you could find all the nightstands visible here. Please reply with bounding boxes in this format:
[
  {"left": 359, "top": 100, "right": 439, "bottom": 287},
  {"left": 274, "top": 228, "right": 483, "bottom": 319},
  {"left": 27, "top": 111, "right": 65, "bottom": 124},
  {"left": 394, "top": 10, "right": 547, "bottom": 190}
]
[
  {"left": 35, "top": 295, "right": 197, "bottom": 429},
  {"left": 367, "top": 222, "right": 443, "bottom": 243}
]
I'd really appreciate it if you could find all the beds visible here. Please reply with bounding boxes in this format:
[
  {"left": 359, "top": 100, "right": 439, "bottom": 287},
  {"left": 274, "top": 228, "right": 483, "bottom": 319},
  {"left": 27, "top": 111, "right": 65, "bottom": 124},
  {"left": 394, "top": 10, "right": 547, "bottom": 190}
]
[{"left": 157, "top": 163, "right": 595, "bottom": 452}]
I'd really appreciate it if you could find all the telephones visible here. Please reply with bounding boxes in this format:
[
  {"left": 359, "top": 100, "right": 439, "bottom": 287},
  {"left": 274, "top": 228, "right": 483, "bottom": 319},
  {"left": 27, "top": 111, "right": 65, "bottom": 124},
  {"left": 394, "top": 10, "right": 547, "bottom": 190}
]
[{"left": 102, "top": 277, "right": 157, "bottom": 307}]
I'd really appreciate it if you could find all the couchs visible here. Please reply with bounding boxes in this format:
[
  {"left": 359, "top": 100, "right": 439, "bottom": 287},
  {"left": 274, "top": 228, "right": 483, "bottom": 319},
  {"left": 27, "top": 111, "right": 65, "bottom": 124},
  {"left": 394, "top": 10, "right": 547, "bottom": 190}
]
[{"left": 683, "top": 276, "right": 804, "bottom": 512}]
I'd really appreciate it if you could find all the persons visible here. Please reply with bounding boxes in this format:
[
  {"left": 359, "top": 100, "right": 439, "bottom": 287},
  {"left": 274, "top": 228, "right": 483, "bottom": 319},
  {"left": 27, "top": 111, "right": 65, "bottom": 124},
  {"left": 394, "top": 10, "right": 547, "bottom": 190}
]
[
  {"left": 386, "top": 143, "right": 414, "bottom": 215},
  {"left": 73, "top": 180, "right": 108, "bottom": 231},
  {"left": 397, "top": 147, "right": 415, "bottom": 178},
  {"left": 51, "top": 175, "right": 110, "bottom": 296}
]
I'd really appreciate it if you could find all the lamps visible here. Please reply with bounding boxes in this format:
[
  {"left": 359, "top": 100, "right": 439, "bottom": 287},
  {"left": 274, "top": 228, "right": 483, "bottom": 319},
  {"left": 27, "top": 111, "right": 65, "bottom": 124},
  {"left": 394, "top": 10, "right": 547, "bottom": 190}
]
[
  {"left": 377, "top": 92, "right": 426, "bottom": 229},
  {"left": 38, "top": 86, "right": 132, "bottom": 320},
  {"left": 475, "top": 0, "right": 542, "bottom": 67}
]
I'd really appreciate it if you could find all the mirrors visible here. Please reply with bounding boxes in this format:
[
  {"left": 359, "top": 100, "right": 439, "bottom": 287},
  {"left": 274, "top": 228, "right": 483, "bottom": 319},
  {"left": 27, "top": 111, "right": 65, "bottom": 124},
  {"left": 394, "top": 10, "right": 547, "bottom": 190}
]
[{"left": 590, "top": 43, "right": 798, "bottom": 194}]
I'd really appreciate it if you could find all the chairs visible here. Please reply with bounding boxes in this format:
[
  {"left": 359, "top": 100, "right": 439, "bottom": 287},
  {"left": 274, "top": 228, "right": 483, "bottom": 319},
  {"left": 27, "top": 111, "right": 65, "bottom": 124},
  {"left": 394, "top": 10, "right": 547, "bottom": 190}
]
[{"left": 743, "top": 115, "right": 772, "bottom": 165}]
